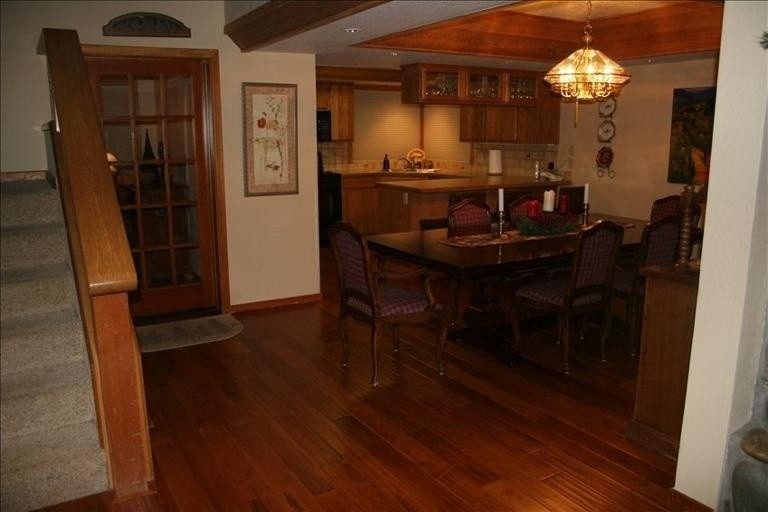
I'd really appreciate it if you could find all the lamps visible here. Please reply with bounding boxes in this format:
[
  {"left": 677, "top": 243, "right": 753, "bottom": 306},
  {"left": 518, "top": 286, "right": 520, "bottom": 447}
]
[{"left": 543, "top": 0, "right": 631, "bottom": 104}]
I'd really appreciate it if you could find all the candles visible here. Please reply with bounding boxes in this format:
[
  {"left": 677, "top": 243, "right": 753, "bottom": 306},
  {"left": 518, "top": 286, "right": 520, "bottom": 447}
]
[
  {"left": 498, "top": 188, "right": 504, "bottom": 212},
  {"left": 584, "top": 184, "right": 590, "bottom": 203}
]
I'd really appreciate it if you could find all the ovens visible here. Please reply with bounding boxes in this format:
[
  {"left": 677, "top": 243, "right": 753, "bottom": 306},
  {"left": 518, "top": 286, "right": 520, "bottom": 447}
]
[{"left": 319, "top": 181, "right": 342, "bottom": 248}]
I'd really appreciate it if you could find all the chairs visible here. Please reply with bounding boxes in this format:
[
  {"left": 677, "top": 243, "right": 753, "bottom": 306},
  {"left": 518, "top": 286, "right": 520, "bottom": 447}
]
[
  {"left": 511, "top": 218, "right": 624, "bottom": 374},
  {"left": 448, "top": 198, "right": 491, "bottom": 237},
  {"left": 329, "top": 222, "right": 452, "bottom": 387},
  {"left": 505, "top": 195, "right": 545, "bottom": 226},
  {"left": 602, "top": 214, "right": 681, "bottom": 359},
  {"left": 650, "top": 195, "right": 701, "bottom": 228}
]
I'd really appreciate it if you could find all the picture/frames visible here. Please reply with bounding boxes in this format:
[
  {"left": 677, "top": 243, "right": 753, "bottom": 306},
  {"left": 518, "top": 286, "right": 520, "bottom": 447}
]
[{"left": 241, "top": 82, "right": 299, "bottom": 197}]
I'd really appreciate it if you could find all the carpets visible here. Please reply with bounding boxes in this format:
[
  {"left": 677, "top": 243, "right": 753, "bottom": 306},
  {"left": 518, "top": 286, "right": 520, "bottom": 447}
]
[{"left": 135, "top": 312, "right": 245, "bottom": 354}]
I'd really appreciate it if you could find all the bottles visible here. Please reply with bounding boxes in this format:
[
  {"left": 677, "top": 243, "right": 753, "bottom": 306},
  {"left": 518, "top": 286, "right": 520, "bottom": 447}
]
[
  {"left": 472, "top": 87, "right": 496, "bottom": 98},
  {"left": 511, "top": 86, "right": 534, "bottom": 100},
  {"left": 383, "top": 154, "right": 390, "bottom": 169}
]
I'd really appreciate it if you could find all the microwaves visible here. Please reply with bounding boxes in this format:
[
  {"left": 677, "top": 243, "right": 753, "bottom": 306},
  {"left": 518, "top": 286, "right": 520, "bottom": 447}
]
[{"left": 316, "top": 111, "right": 331, "bottom": 143}]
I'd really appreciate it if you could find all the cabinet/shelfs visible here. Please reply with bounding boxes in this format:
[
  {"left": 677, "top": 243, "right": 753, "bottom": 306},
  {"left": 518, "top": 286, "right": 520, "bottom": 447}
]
[
  {"left": 400, "top": 63, "right": 542, "bottom": 107},
  {"left": 460, "top": 71, "right": 560, "bottom": 144},
  {"left": 342, "top": 175, "right": 380, "bottom": 227},
  {"left": 629, "top": 260, "right": 698, "bottom": 441}
]
